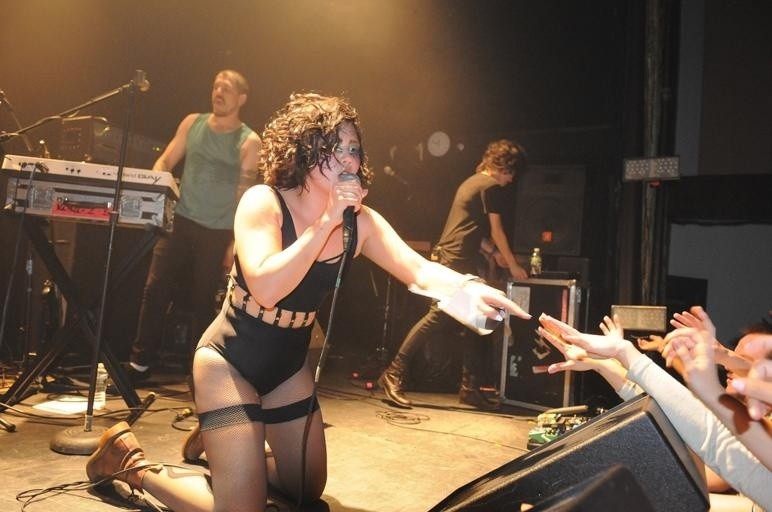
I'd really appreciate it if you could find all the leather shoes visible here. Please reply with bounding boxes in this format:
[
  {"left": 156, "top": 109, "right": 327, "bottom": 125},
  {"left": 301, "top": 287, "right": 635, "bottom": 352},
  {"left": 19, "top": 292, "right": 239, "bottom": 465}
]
[{"left": 106, "top": 362, "right": 152, "bottom": 394}]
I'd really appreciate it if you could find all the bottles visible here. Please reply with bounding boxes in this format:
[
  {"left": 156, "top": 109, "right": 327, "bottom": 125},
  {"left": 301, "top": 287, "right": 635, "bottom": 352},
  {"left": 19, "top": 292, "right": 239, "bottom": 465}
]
[
  {"left": 530, "top": 247, "right": 542, "bottom": 274},
  {"left": 93, "top": 363, "right": 109, "bottom": 411}
]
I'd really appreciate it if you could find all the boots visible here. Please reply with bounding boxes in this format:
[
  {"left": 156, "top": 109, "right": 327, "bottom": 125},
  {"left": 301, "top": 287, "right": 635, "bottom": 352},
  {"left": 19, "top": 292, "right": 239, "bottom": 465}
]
[
  {"left": 183, "top": 425, "right": 205, "bottom": 461},
  {"left": 377, "top": 361, "right": 412, "bottom": 407},
  {"left": 459, "top": 365, "right": 501, "bottom": 411},
  {"left": 86, "top": 421, "right": 163, "bottom": 495}
]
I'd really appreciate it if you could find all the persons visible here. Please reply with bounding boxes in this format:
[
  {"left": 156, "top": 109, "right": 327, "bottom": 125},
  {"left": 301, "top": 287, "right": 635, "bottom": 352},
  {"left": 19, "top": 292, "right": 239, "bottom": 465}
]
[
  {"left": 103, "top": 69, "right": 266, "bottom": 385},
  {"left": 83, "top": 88, "right": 532, "bottom": 510},
  {"left": 379, "top": 135, "right": 528, "bottom": 409}
]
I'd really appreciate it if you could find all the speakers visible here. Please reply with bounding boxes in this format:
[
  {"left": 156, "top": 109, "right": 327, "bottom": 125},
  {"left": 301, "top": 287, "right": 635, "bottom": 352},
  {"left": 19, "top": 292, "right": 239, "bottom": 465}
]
[
  {"left": 510, "top": 166, "right": 609, "bottom": 259},
  {"left": 427, "top": 391, "right": 711, "bottom": 512}
]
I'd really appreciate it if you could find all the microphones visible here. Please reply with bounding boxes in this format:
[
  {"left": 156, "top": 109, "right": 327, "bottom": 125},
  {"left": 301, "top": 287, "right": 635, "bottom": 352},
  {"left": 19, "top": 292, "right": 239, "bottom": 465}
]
[
  {"left": 134, "top": 69, "right": 151, "bottom": 94},
  {"left": 336, "top": 170, "right": 362, "bottom": 256}
]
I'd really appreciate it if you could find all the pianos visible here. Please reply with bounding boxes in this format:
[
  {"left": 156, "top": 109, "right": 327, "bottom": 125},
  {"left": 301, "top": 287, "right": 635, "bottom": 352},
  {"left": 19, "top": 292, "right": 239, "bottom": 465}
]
[{"left": 2, "top": 154, "right": 181, "bottom": 202}]
